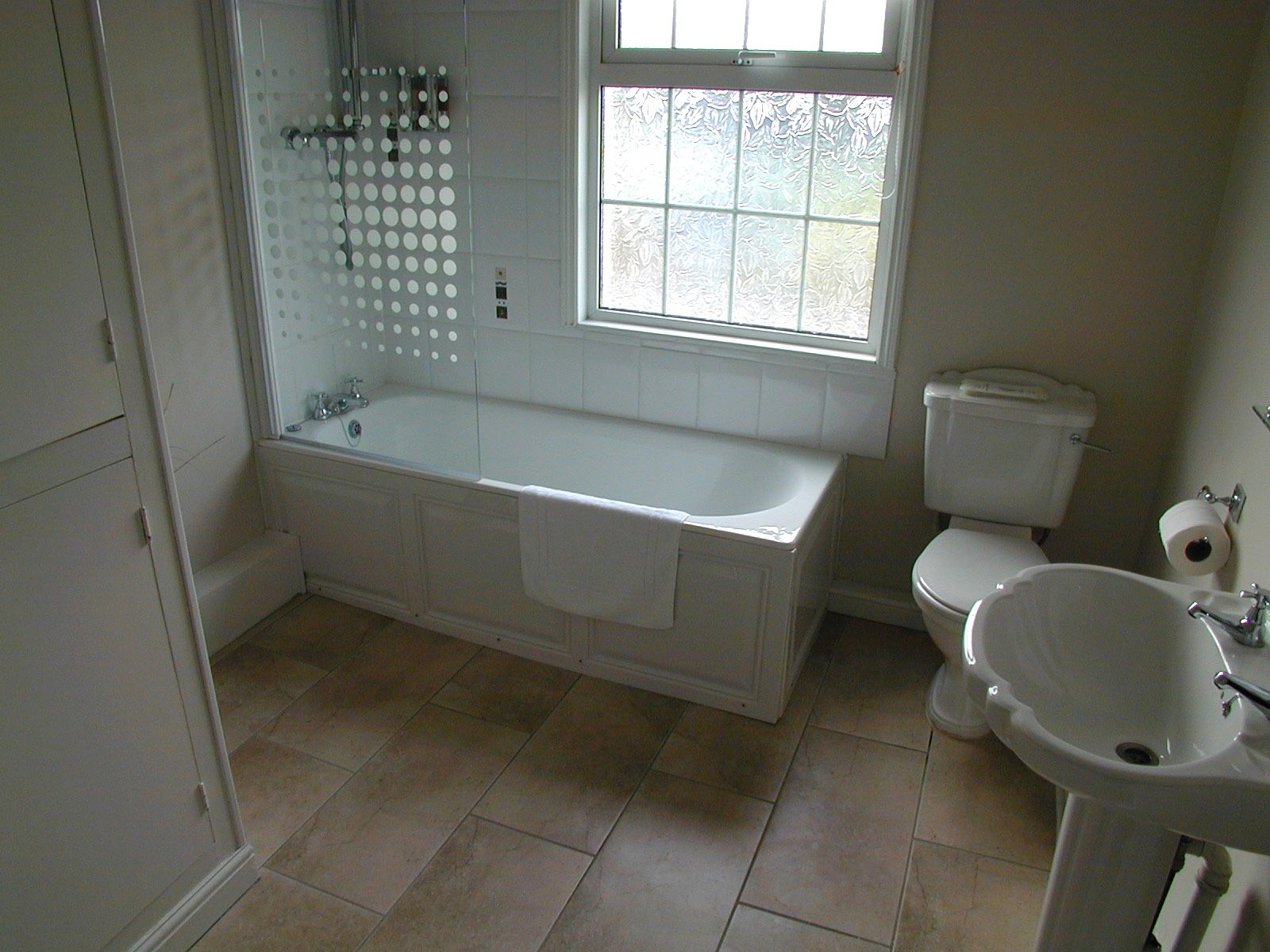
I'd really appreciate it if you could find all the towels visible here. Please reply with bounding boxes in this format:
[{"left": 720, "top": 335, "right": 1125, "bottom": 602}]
[{"left": 516, "top": 486, "right": 689, "bottom": 630}]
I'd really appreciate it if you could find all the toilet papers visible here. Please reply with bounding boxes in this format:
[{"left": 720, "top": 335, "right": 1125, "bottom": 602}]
[{"left": 1159, "top": 496, "right": 1233, "bottom": 579}]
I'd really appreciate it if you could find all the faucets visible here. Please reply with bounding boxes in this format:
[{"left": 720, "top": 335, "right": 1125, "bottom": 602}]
[
  {"left": 1186, "top": 581, "right": 1270, "bottom": 646},
  {"left": 325, "top": 378, "right": 371, "bottom": 411}
]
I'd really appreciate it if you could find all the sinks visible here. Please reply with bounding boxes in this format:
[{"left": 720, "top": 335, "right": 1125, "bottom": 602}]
[{"left": 961, "top": 561, "right": 1243, "bottom": 804}]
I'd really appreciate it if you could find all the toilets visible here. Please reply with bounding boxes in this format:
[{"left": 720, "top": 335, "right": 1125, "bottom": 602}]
[{"left": 907, "top": 368, "right": 1101, "bottom": 739}]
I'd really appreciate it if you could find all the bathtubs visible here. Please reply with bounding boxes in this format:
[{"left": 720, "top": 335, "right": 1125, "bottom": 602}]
[{"left": 272, "top": 383, "right": 850, "bottom": 732}]
